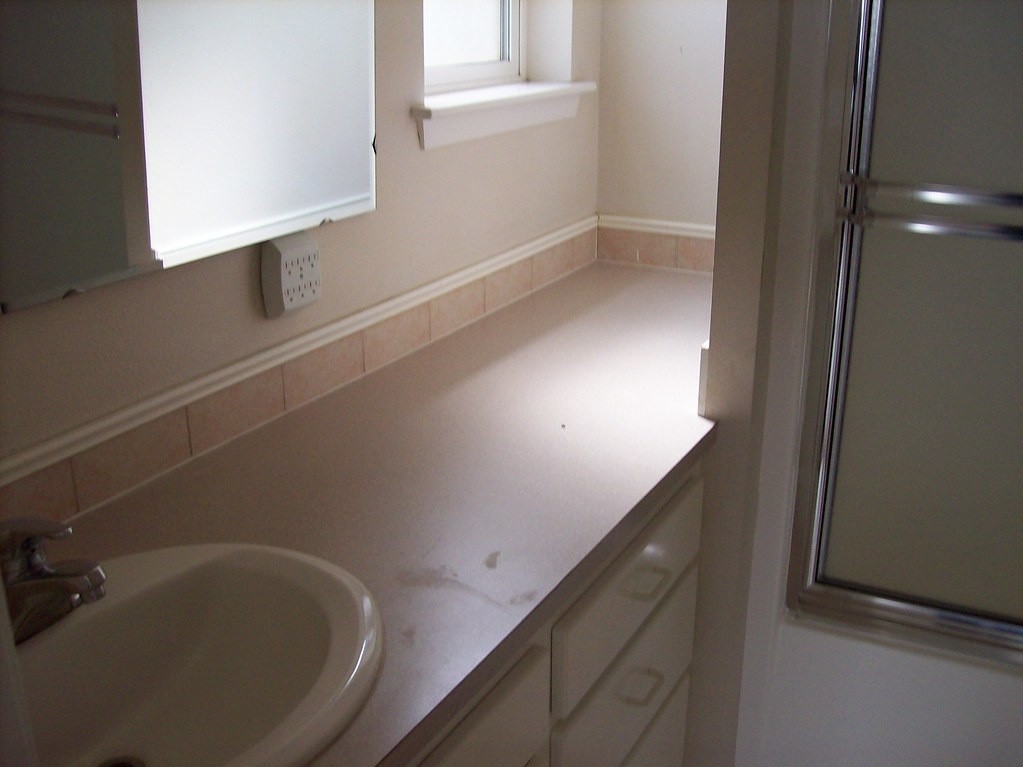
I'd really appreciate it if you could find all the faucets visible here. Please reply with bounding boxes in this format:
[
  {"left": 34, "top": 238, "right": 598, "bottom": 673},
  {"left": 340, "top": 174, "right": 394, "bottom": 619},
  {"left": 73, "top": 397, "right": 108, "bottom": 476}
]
[{"left": 0, "top": 517, "right": 109, "bottom": 642}]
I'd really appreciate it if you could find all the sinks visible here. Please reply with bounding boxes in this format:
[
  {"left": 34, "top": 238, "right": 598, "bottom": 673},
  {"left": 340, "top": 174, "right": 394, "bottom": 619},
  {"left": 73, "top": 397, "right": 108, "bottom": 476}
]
[{"left": 14, "top": 541, "right": 388, "bottom": 767}]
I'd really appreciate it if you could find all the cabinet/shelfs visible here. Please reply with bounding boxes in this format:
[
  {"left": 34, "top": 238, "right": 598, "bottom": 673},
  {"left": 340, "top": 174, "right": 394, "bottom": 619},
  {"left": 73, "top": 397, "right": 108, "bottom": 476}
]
[{"left": 404, "top": 477, "right": 705, "bottom": 767}]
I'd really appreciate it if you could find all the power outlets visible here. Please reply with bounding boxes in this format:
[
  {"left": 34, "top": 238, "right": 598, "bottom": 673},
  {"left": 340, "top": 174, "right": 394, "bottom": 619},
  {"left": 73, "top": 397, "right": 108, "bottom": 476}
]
[{"left": 260, "top": 237, "right": 323, "bottom": 320}]
